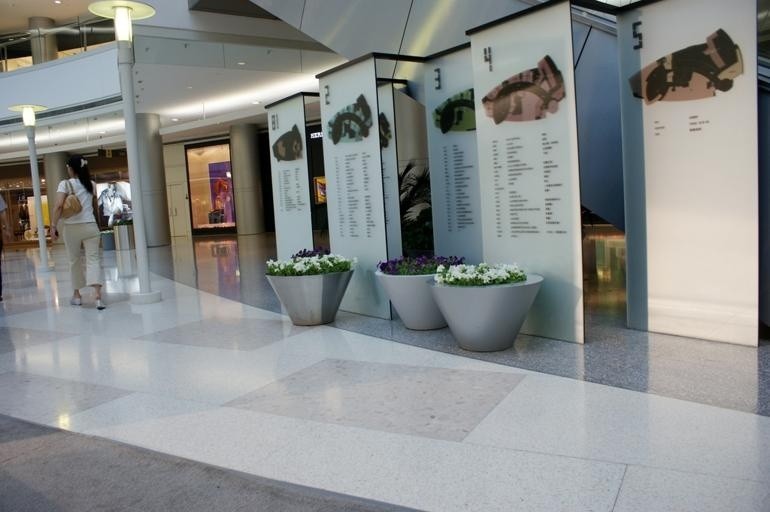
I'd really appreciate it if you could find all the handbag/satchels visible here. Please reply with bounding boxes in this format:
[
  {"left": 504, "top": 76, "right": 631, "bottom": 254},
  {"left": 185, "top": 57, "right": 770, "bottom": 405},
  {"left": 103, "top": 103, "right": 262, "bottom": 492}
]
[{"left": 61, "top": 179, "right": 82, "bottom": 218}]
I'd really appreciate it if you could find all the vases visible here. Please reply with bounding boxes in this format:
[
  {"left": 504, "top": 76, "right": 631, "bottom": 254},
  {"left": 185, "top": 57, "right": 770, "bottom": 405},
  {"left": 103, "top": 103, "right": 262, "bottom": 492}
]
[
  {"left": 265, "top": 268, "right": 352, "bottom": 327},
  {"left": 370, "top": 258, "right": 450, "bottom": 333},
  {"left": 429, "top": 275, "right": 550, "bottom": 354}
]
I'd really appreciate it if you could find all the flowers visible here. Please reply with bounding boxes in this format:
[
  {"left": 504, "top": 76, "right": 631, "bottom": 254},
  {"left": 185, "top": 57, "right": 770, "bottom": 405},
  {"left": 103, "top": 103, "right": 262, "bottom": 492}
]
[
  {"left": 376, "top": 253, "right": 466, "bottom": 273},
  {"left": 266, "top": 249, "right": 356, "bottom": 275},
  {"left": 436, "top": 262, "right": 532, "bottom": 286}
]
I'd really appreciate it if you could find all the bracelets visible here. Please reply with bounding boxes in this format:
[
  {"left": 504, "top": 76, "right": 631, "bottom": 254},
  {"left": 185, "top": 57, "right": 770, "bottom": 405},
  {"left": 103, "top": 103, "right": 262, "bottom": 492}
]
[{"left": 52, "top": 222, "right": 57, "bottom": 227}]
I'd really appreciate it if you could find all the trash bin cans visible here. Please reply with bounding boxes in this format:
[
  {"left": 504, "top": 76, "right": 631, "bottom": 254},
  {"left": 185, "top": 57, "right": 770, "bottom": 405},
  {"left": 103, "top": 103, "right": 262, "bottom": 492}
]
[{"left": 100, "top": 230, "right": 115, "bottom": 250}]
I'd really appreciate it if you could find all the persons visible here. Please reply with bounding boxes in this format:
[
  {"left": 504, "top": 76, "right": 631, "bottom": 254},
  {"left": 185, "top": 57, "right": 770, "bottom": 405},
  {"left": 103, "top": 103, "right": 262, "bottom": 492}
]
[
  {"left": 0, "top": 194, "right": 8, "bottom": 301},
  {"left": 102, "top": 182, "right": 127, "bottom": 214},
  {"left": 51, "top": 154, "right": 105, "bottom": 310}
]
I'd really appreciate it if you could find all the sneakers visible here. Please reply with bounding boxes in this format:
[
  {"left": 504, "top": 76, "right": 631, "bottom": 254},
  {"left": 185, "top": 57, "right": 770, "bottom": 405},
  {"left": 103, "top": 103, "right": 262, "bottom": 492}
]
[
  {"left": 70, "top": 298, "right": 81, "bottom": 305},
  {"left": 96, "top": 300, "right": 106, "bottom": 310}
]
